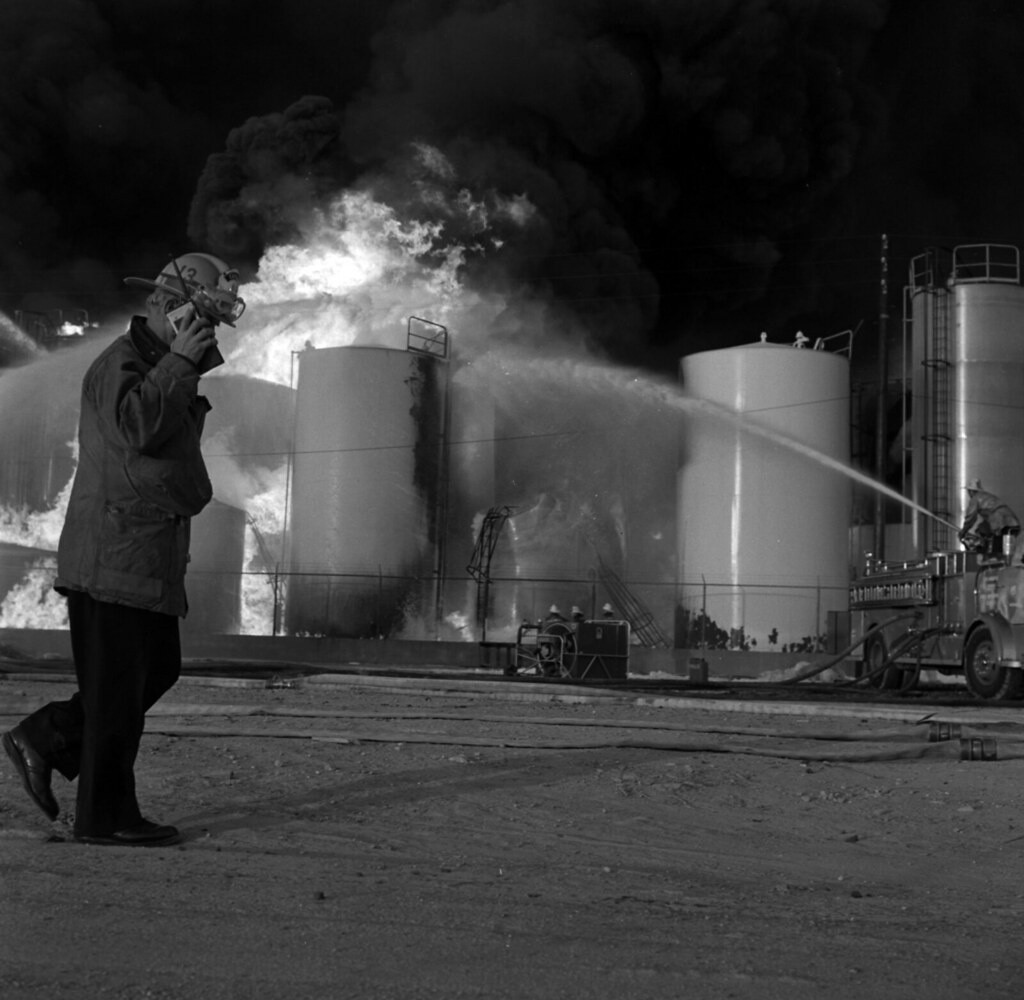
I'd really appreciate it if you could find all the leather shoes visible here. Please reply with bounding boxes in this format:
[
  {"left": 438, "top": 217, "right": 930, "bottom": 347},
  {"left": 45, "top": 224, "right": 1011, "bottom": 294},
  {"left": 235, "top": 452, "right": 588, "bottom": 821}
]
[
  {"left": 2, "top": 729, "right": 60, "bottom": 823},
  {"left": 75, "top": 815, "right": 180, "bottom": 847}
]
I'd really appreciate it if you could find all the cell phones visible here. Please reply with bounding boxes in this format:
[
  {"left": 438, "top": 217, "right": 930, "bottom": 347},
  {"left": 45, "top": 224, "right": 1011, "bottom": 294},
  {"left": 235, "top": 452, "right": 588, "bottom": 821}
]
[{"left": 167, "top": 301, "right": 204, "bottom": 337}]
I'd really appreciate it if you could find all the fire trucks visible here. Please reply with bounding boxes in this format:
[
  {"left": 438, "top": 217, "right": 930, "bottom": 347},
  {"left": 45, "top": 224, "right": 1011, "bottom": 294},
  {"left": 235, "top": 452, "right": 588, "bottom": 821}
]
[{"left": 844, "top": 526, "right": 1024, "bottom": 702}]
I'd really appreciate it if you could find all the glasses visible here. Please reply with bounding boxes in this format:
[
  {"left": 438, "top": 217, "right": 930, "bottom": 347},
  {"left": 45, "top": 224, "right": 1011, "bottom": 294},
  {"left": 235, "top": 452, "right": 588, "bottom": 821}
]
[{"left": 194, "top": 283, "right": 246, "bottom": 322}]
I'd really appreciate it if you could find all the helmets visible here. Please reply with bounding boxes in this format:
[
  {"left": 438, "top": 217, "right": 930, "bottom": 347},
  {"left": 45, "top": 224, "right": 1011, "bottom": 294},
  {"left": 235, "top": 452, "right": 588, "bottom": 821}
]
[{"left": 123, "top": 252, "right": 240, "bottom": 328}]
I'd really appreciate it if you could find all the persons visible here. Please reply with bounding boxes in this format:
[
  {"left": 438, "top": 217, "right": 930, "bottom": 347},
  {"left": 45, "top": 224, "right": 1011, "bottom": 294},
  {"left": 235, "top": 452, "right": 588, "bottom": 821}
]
[
  {"left": 958, "top": 479, "right": 1020, "bottom": 563},
  {"left": 541, "top": 602, "right": 617, "bottom": 621},
  {"left": 0, "top": 251, "right": 248, "bottom": 846}
]
